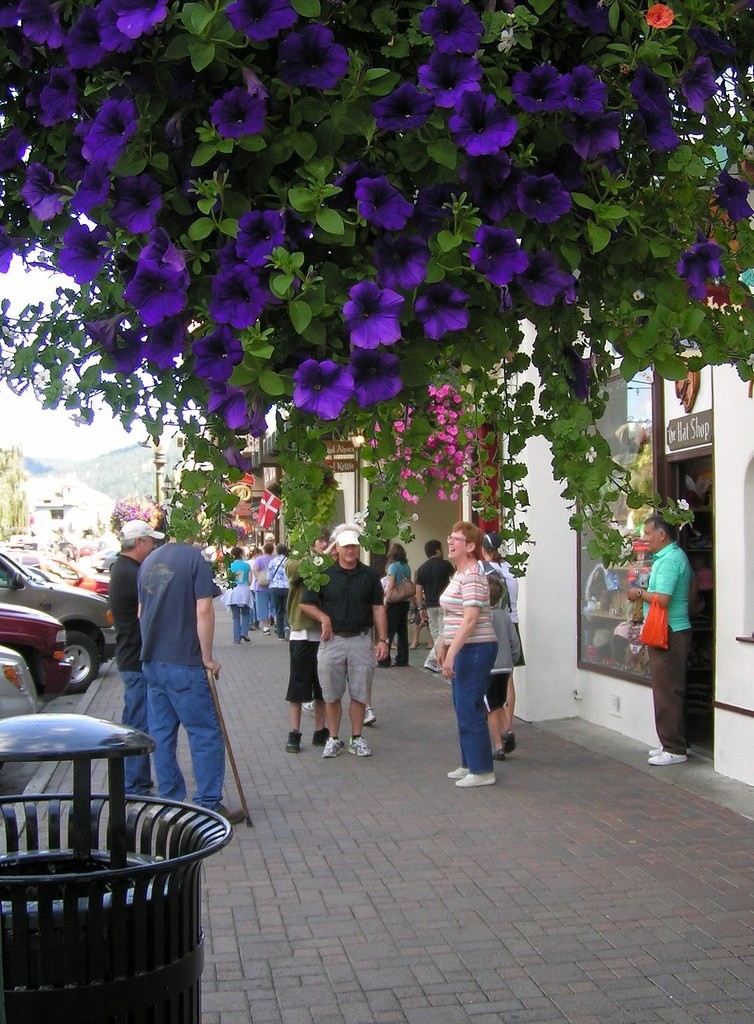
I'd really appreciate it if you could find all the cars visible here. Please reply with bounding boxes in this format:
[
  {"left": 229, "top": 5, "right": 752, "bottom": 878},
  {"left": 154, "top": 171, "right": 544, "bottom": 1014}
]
[
  {"left": 0, "top": 646, "right": 37, "bottom": 770},
  {"left": 0, "top": 549, "right": 112, "bottom": 595},
  {"left": 0, "top": 535, "right": 101, "bottom": 564},
  {"left": 0, "top": 601, "right": 73, "bottom": 702},
  {"left": 91, "top": 550, "right": 121, "bottom": 573},
  {"left": 0, "top": 552, "right": 118, "bottom": 695}
]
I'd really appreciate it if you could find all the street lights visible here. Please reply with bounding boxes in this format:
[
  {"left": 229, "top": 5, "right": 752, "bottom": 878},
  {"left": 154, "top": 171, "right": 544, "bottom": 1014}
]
[{"left": 152, "top": 427, "right": 171, "bottom": 507}]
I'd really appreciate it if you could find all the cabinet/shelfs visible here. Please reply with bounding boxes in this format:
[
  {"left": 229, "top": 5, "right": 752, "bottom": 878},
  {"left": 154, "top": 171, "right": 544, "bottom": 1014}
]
[
  {"left": 583, "top": 566, "right": 644, "bottom": 626},
  {"left": 679, "top": 505, "right": 714, "bottom": 671}
]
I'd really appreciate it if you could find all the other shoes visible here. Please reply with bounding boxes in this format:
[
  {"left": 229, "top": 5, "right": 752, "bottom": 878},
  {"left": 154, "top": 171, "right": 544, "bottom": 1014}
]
[
  {"left": 455, "top": 774, "right": 496, "bottom": 787},
  {"left": 214, "top": 805, "right": 245, "bottom": 824},
  {"left": 448, "top": 768, "right": 469, "bottom": 779},
  {"left": 240, "top": 623, "right": 290, "bottom": 642},
  {"left": 313, "top": 728, "right": 329, "bottom": 744},
  {"left": 285, "top": 733, "right": 301, "bottom": 752},
  {"left": 493, "top": 749, "right": 505, "bottom": 760},
  {"left": 503, "top": 731, "right": 515, "bottom": 754}
]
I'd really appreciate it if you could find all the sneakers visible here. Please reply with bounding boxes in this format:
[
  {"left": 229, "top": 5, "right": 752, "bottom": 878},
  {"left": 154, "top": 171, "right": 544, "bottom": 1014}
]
[
  {"left": 322, "top": 738, "right": 343, "bottom": 757},
  {"left": 302, "top": 700, "right": 315, "bottom": 711},
  {"left": 424, "top": 664, "right": 442, "bottom": 673},
  {"left": 648, "top": 752, "right": 688, "bottom": 765},
  {"left": 649, "top": 747, "right": 692, "bottom": 755},
  {"left": 348, "top": 736, "right": 371, "bottom": 756},
  {"left": 363, "top": 708, "right": 376, "bottom": 726}
]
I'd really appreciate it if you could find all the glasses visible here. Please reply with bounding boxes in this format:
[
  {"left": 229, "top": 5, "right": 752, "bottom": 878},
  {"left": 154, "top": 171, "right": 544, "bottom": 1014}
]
[{"left": 447, "top": 536, "right": 472, "bottom": 542}]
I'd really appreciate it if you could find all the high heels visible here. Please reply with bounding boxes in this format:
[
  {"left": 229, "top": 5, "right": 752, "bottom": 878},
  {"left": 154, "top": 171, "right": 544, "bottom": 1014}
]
[
  {"left": 426, "top": 643, "right": 433, "bottom": 649},
  {"left": 410, "top": 642, "right": 420, "bottom": 650}
]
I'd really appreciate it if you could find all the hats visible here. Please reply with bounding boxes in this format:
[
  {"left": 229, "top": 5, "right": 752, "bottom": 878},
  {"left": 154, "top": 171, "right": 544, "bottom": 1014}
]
[
  {"left": 119, "top": 519, "right": 165, "bottom": 541},
  {"left": 336, "top": 530, "right": 360, "bottom": 547}
]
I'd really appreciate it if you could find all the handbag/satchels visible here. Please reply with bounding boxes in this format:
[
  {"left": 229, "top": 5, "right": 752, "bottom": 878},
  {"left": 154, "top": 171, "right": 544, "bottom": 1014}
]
[
  {"left": 639, "top": 593, "right": 669, "bottom": 650},
  {"left": 385, "top": 564, "right": 415, "bottom": 603},
  {"left": 257, "top": 557, "right": 270, "bottom": 585}
]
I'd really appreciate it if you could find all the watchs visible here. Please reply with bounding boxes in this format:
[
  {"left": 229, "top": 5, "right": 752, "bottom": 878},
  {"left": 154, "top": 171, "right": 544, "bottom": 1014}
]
[
  {"left": 379, "top": 638, "right": 390, "bottom": 645},
  {"left": 636, "top": 589, "right": 644, "bottom": 600}
]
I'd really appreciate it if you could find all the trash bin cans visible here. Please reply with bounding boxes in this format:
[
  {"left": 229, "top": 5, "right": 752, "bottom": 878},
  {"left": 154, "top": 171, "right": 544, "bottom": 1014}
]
[{"left": 0, "top": 713, "right": 234, "bottom": 1024}]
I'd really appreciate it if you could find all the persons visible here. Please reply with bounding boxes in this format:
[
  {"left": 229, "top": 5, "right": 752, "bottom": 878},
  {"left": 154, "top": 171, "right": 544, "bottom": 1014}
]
[
  {"left": 108, "top": 519, "right": 165, "bottom": 796},
  {"left": 136, "top": 510, "right": 244, "bottom": 824},
  {"left": 436, "top": 522, "right": 499, "bottom": 788},
  {"left": 379, "top": 534, "right": 528, "bottom": 760},
  {"left": 626, "top": 515, "right": 697, "bottom": 766},
  {"left": 221, "top": 523, "right": 390, "bottom": 758}
]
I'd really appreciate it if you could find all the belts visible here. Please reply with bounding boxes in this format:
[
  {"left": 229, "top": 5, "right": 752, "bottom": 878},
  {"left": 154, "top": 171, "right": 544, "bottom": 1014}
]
[{"left": 334, "top": 632, "right": 367, "bottom": 637}]
